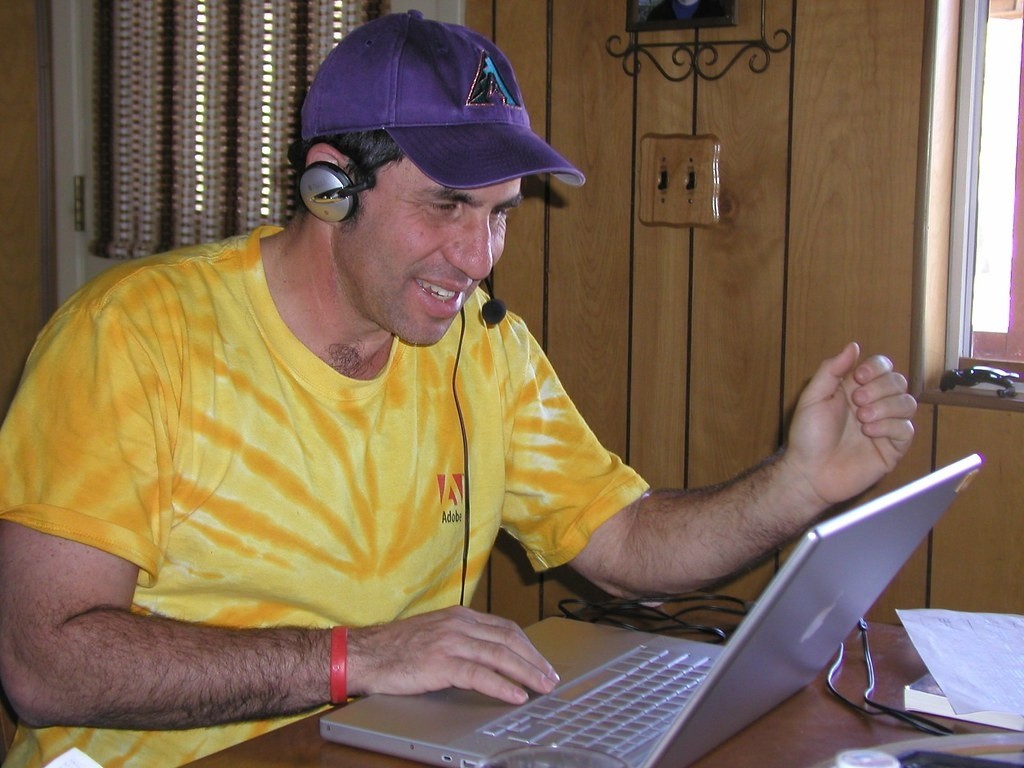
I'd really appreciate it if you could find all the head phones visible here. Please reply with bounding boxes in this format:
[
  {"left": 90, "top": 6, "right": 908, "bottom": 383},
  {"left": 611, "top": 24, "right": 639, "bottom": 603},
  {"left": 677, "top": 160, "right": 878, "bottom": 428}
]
[{"left": 298, "top": 161, "right": 375, "bottom": 223}]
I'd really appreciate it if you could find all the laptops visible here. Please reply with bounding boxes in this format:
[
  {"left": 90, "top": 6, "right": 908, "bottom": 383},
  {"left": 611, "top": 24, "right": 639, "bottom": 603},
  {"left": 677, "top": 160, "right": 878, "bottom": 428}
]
[{"left": 319, "top": 454, "right": 983, "bottom": 768}]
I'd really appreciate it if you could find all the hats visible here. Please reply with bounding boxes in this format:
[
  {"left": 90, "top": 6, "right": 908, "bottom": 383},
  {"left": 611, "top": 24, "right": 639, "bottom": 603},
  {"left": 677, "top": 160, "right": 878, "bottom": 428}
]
[{"left": 300, "top": 10, "right": 586, "bottom": 189}]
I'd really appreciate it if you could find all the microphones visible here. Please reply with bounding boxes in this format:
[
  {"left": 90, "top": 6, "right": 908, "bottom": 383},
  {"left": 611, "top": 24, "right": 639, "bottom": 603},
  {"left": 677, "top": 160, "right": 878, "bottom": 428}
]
[{"left": 482, "top": 271, "right": 507, "bottom": 324}]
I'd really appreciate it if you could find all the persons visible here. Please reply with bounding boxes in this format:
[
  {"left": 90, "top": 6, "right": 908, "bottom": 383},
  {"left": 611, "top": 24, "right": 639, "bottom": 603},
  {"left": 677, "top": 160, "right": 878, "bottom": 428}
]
[{"left": 0, "top": 7, "right": 924, "bottom": 767}]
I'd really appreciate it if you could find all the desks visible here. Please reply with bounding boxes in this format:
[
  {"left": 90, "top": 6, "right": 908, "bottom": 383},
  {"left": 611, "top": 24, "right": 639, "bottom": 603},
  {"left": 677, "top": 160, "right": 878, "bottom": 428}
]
[{"left": 171, "top": 590, "right": 1019, "bottom": 768}]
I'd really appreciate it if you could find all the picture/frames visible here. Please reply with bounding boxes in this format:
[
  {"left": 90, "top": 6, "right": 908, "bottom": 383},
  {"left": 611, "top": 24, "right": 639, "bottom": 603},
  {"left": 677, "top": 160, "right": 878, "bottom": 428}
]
[{"left": 625, "top": 0, "right": 740, "bottom": 33}]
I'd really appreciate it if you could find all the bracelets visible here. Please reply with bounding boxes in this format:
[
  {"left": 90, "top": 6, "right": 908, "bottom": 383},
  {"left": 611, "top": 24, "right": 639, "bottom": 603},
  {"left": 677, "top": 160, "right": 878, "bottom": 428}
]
[{"left": 328, "top": 624, "right": 350, "bottom": 708}]
